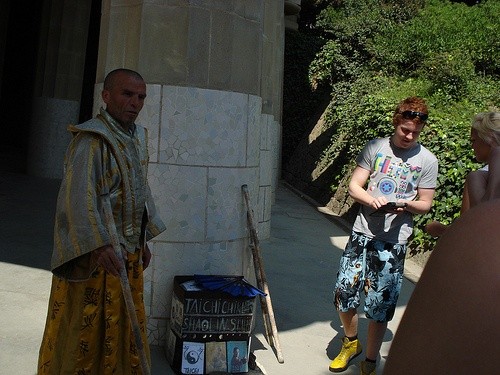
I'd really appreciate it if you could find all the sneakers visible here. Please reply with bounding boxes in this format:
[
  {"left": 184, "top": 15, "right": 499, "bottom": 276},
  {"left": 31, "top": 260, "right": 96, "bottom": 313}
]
[
  {"left": 329, "top": 337, "right": 362, "bottom": 372},
  {"left": 360, "top": 360, "right": 376, "bottom": 375}
]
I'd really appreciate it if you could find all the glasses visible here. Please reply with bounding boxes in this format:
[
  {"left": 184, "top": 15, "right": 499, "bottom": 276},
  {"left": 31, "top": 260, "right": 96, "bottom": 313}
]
[{"left": 399, "top": 110, "right": 428, "bottom": 122}]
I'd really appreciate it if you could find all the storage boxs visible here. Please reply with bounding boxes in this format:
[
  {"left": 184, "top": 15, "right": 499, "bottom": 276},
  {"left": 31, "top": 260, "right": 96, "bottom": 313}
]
[{"left": 164, "top": 275, "right": 255, "bottom": 375}]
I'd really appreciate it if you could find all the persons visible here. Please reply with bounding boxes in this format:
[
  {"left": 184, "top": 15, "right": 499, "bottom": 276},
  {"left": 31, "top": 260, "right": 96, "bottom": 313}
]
[
  {"left": 38, "top": 68, "right": 167, "bottom": 375},
  {"left": 231, "top": 346, "right": 246, "bottom": 373},
  {"left": 381, "top": 108, "right": 500, "bottom": 375},
  {"left": 328, "top": 97, "right": 438, "bottom": 375}
]
